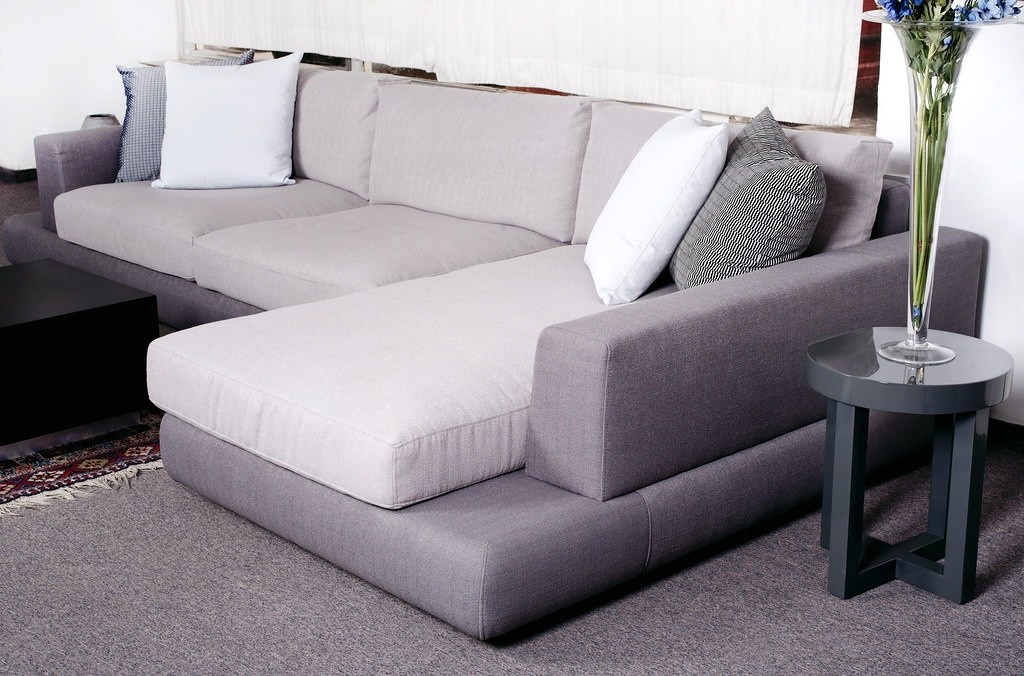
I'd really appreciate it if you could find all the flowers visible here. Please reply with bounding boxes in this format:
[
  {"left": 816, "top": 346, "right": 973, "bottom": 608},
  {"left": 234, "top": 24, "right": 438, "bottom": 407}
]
[{"left": 874, "top": 0, "right": 1022, "bottom": 21}]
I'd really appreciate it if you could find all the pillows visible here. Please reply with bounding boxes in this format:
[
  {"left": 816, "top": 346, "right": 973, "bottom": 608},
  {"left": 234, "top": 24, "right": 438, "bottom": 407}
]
[
  {"left": 580, "top": 107, "right": 732, "bottom": 308},
  {"left": 112, "top": 46, "right": 255, "bottom": 183},
  {"left": 151, "top": 50, "right": 305, "bottom": 189},
  {"left": 669, "top": 107, "right": 830, "bottom": 291}
]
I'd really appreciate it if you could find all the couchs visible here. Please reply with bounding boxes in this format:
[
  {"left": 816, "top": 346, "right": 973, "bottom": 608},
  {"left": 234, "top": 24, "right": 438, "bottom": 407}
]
[{"left": 0, "top": 49, "right": 991, "bottom": 650}]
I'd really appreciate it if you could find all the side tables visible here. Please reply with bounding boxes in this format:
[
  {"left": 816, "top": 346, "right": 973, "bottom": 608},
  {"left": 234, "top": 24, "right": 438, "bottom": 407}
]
[{"left": 802, "top": 324, "right": 1016, "bottom": 606}]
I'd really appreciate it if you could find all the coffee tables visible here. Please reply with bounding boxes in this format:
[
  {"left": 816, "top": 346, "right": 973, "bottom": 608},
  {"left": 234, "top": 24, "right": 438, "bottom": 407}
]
[{"left": 0, "top": 256, "right": 165, "bottom": 448}]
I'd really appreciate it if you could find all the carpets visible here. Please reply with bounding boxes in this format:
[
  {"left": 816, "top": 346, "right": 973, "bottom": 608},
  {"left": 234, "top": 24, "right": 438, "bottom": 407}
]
[{"left": 0, "top": 403, "right": 165, "bottom": 515}]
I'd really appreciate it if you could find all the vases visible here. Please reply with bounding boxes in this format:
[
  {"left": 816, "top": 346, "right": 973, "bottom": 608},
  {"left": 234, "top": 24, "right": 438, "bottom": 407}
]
[{"left": 852, "top": 7, "right": 1024, "bottom": 368}]
